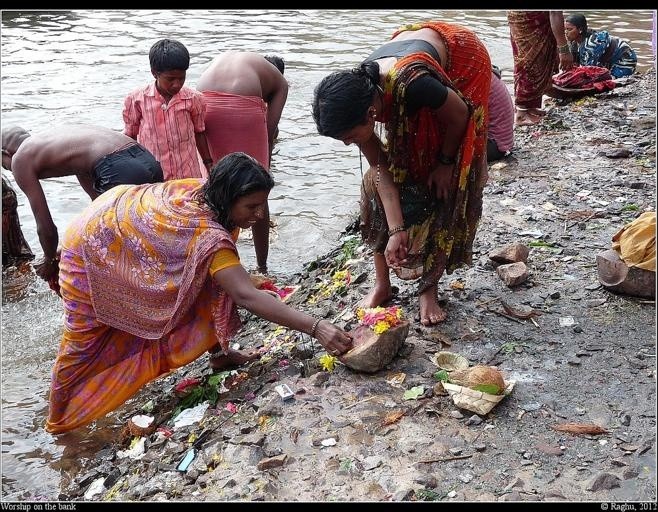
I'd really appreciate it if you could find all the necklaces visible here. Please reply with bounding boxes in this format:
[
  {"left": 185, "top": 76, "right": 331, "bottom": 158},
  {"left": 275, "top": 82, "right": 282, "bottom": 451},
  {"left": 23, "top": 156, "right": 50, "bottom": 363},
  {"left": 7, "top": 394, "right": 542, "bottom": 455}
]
[
  {"left": 159, "top": 89, "right": 172, "bottom": 110},
  {"left": 358, "top": 94, "right": 383, "bottom": 210}
]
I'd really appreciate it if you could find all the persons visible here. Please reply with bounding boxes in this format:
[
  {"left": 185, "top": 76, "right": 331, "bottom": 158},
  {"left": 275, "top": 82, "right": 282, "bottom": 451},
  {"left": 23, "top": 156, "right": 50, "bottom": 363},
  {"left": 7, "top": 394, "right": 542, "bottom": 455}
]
[
  {"left": 43, "top": 152, "right": 354, "bottom": 434},
  {"left": 121, "top": 38, "right": 209, "bottom": 181},
  {"left": 486, "top": 63, "right": 515, "bottom": 162},
  {"left": 309, "top": 20, "right": 492, "bottom": 326},
  {"left": 563, "top": 12, "right": 638, "bottom": 79},
  {"left": 2, "top": 121, "right": 165, "bottom": 283},
  {"left": 2, "top": 177, "right": 36, "bottom": 273},
  {"left": 507, "top": 11, "right": 574, "bottom": 127},
  {"left": 193, "top": 48, "right": 288, "bottom": 266}
]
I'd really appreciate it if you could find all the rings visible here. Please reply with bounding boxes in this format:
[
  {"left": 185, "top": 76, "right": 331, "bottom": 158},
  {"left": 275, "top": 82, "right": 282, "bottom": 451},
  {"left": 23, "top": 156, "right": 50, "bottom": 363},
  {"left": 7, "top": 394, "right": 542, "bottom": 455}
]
[{"left": 331, "top": 348, "right": 338, "bottom": 354}]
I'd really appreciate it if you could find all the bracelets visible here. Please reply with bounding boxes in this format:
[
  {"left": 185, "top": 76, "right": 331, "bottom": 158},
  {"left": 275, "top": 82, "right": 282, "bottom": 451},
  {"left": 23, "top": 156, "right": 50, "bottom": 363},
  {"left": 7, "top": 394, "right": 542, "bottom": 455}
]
[
  {"left": 387, "top": 225, "right": 407, "bottom": 237},
  {"left": 310, "top": 318, "right": 322, "bottom": 338},
  {"left": 46, "top": 256, "right": 59, "bottom": 265},
  {"left": 437, "top": 154, "right": 459, "bottom": 168},
  {"left": 556, "top": 43, "right": 570, "bottom": 55}
]
